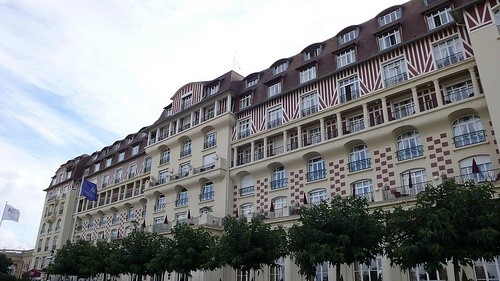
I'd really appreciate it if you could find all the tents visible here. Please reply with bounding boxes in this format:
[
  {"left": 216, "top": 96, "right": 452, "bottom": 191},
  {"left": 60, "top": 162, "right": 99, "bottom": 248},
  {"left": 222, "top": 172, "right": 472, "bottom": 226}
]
[{"left": 27, "top": 268, "right": 48, "bottom": 281}]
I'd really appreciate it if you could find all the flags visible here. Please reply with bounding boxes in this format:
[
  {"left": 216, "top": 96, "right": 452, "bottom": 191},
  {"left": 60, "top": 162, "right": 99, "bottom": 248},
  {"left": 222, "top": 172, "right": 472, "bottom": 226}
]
[
  {"left": 269, "top": 201, "right": 275, "bottom": 212},
  {"left": 236, "top": 208, "right": 238, "bottom": 218},
  {"left": 409, "top": 170, "right": 412, "bottom": 189},
  {"left": 304, "top": 192, "right": 308, "bottom": 205},
  {"left": 90, "top": 229, "right": 120, "bottom": 242},
  {"left": 141, "top": 220, "right": 146, "bottom": 228},
  {"left": 353, "top": 183, "right": 356, "bottom": 198},
  {"left": 472, "top": 158, "right": 480, "bottom": 173},
  {"left": 187, "top": 209, "right": 190, "bottom": 219},
  {"left": 2, "top": 204, "right": 20, "bottom": 222},
  {"left": 163, "top": 215, "right": 168, "bottom": 224},
  {"left": 79, "top": 179, "right": 98, "bottom": 201}
]
